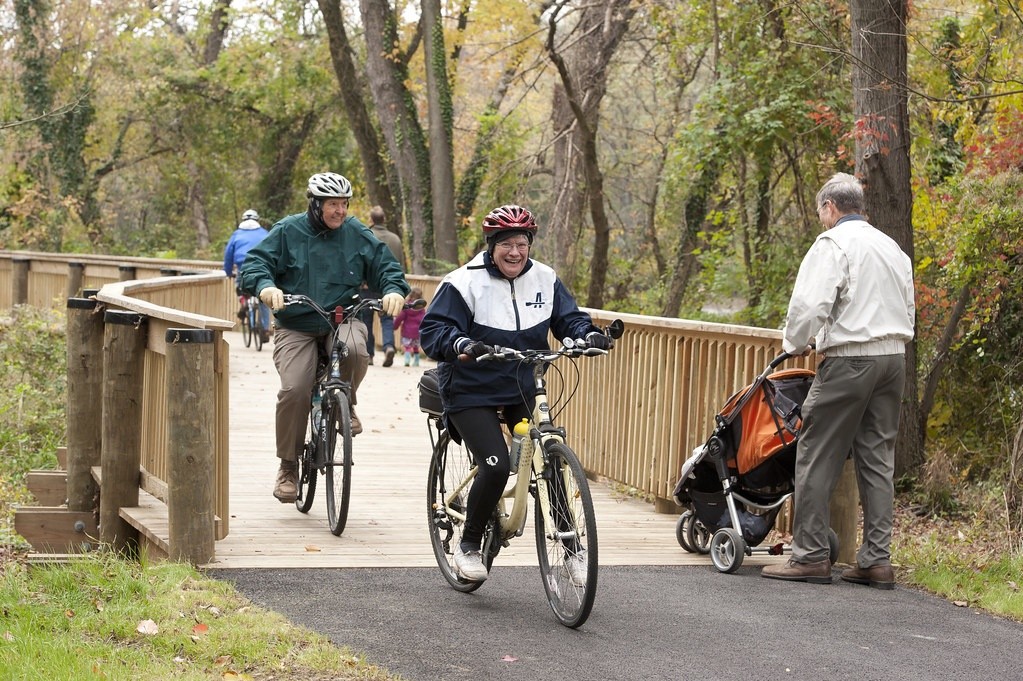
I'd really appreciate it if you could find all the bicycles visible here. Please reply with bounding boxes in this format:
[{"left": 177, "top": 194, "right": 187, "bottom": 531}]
[
  {"left": 229, "top": 273, "right": 270, "bottom": 351},
  {"left": 262, "top": 292, "right": 431, "bottom": 537},
  {"left": 421, "top": 318, "right": 628, "bottom": 630}
]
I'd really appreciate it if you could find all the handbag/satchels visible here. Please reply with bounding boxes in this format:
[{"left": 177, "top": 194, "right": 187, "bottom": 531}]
[{"left": 417, "top": 368, "right": 444, "bottom": 416}]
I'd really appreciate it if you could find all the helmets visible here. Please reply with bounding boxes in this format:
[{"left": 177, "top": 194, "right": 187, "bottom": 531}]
[
  {"left": 242, "top": 209, "right": 259, "bottom": 220},
  {"left": 306, "top": 172, "right": 353, "bottom": 199},
  {"left": 482, "top": 205, "right": 538, "bottom": 238}
]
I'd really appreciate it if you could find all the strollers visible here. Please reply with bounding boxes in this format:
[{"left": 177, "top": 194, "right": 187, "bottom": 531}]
[{"left": 671, "top": 341, "right": 845, "bottom": 573}]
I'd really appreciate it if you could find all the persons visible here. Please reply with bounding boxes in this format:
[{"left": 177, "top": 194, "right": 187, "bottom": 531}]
[
  {"left": 224, "top": 209, "right": 270, "bottom": 343},
  {"left": 418, "top": 204, "right": 610, "bottom": 588},
  {"left": 392, "top": 288, "right": 427, "bottom": 367},
  {"left": 760, "top": 172, "right": 915, "bottom": 590},
  {"left": 361, "top": 205, "right": 406, "bottom": 368},
  {"left": 242, "top": 172, "right": 411, "bottom": 502}
]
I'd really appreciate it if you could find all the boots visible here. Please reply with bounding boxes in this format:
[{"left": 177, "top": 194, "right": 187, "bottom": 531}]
[
  {"left": 413, "top": 352, "right": 420, "bottom": 366},
  {"left": 404, "top": 351, "right": 410, "bottom": 366}
]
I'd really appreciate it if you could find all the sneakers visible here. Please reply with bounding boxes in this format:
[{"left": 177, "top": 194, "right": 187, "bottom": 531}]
[
  {"left": 561, "top": 549, "right": 587, "bottom": 587},
  {"left": 339, "top": 406, "right": 362, "bottom": 436},
  {"left": 273, "top": 469, "right": 298, "bottom": 503},
  {"left": 453, "top": 542, "right": 488, "bottom": 582}
]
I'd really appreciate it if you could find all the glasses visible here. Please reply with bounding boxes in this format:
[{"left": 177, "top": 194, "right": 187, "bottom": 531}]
[
  {"left": 815, "top": 200, "right": 832, "bottom": 218},
  {"left": 495, "top": 242, "right": 532, "bottom": 249}
]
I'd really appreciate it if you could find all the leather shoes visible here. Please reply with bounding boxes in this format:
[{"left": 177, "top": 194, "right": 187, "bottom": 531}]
[
  {"left": 841, "top": 563, "right": 895, "bottom": 590},
  {"left": 761, "top": 559, "right": 832, "bottom": 584}
]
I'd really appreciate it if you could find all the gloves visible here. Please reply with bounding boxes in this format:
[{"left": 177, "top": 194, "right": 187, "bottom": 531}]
[
  {"left": 584, "top": 331, "right": 609, "bottom": 357},
  {"left": 463, "top": 341, "right": 494, "bottom": 361},
  {"left": 260, "top": 286, "right": 286, "bottom": 314},
  {"left": 379, "top": 292, "right": 405, "bottom": 317}
]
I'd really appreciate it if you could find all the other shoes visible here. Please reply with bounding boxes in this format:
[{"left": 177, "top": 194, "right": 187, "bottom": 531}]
[
  {"left": 368, "top": 353, "right": 373, "bottom": 364},
  {"left": 384, "top": 346, "right": 395, "bottom": 367},
  {"left": 238, "top": 306, "right": 249, "bottom": 320},
  {"left": 263, "top": 331, "right": 269, "bottom": 342}
]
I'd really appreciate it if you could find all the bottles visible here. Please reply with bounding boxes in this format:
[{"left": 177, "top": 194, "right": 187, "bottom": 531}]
[
  {"left": 509, "top": 417, "right": 530, "bottom": 472},
  {"left": 311, "top": 384, "right": 322, "bottom": 436}
]
